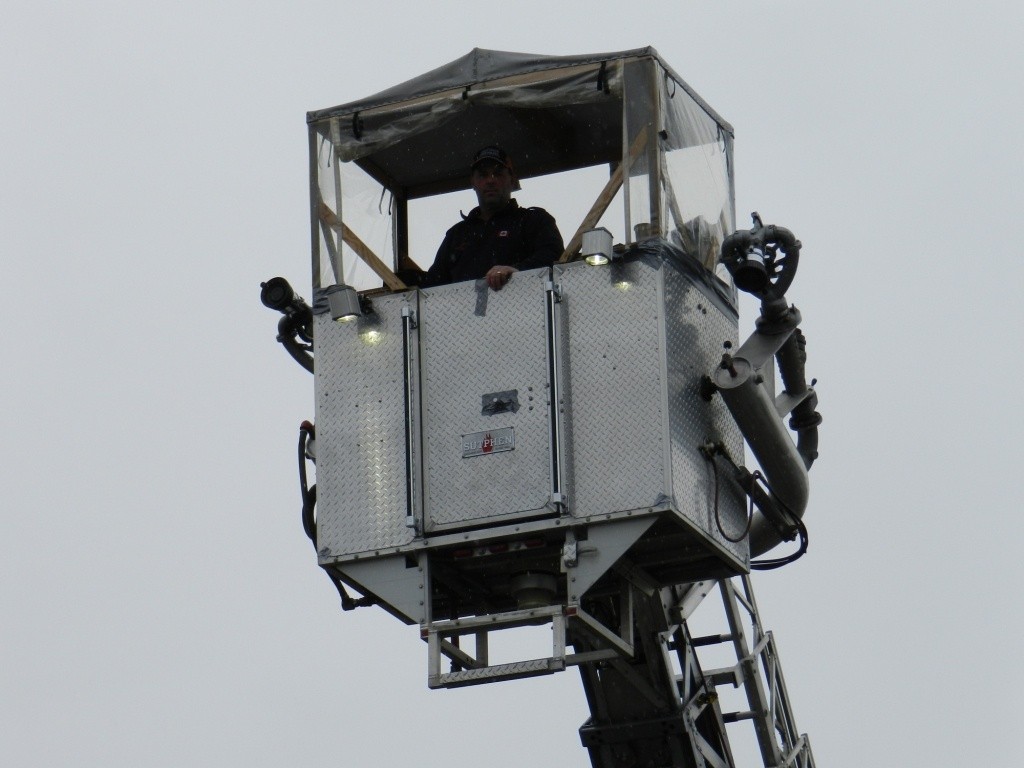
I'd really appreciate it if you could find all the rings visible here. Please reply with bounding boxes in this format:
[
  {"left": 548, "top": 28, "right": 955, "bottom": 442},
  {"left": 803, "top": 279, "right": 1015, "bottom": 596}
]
[{"left": 497, "top": 270, "right": 501, "bottom": 274}]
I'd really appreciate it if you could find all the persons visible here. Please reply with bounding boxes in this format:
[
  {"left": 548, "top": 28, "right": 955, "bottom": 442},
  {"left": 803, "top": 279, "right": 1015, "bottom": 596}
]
[{"left": 422, "top": 146, "right": 565, "bottom": 290}]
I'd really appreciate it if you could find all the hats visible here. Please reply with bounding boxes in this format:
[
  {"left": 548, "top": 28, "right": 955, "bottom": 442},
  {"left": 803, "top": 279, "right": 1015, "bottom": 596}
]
[{"left": 470, "top": 146, "right": 512, "bottom": 171}]
